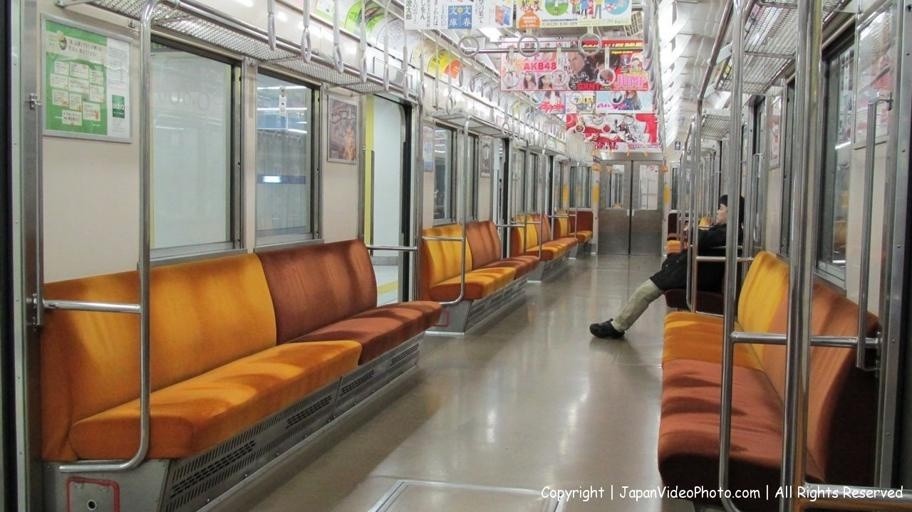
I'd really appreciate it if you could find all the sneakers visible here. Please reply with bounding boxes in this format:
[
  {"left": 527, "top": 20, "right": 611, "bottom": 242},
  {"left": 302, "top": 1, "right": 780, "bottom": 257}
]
[{"left": 589, "top": 318, "right": 625, "bottom": 338}]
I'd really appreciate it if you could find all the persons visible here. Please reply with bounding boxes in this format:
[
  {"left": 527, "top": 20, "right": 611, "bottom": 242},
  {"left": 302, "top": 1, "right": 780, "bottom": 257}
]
[
  {"left": 618, "top": 89, "right": 642, "bottom": 111},
  {"left": 538, "top": 74, "right": 552, "bottom": 90},
  {"left": 567, "top": 50, "right": 600, "bottom": 90},
  {"left": 589, "top": 192, "right": 744, "bottom": 340},
  {"left": 523, "top": 70, "right": 536, "bottom": 89}
]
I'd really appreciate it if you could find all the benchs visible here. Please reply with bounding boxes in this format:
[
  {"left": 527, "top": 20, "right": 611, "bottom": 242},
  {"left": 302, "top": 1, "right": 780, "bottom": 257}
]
[
  {"left": 658, "top": 250, "right": 880, "bottom": 512},
  {"left": 36, "top": 209, "right": 595, "bottom": 463},
  {"left": 665, "top": 218, "right": 712, "bottom": 256}
]
